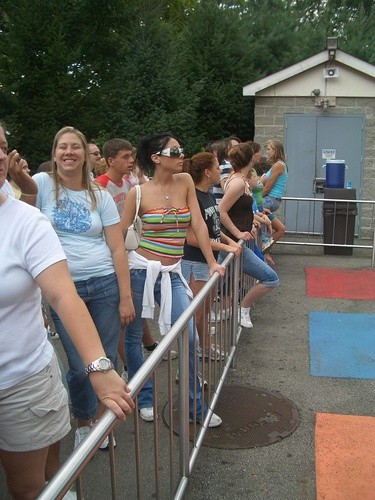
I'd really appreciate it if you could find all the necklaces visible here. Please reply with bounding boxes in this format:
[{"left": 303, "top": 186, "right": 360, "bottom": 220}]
[
  {"left": 154, "top": 178, "right": 174, "bottom": 199},
  {"left": 239, "top": 172, "right": 248, "bottom": 182}
]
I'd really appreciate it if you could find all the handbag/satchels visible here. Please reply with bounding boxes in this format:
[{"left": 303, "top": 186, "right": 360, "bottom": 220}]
[{"left": 124, "top": 184, "right": 143, "bottom": 250}]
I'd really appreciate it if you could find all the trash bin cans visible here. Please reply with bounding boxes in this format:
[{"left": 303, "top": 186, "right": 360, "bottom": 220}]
[{"left": 323, "top": 188, "right": 356, "bottom": 255}]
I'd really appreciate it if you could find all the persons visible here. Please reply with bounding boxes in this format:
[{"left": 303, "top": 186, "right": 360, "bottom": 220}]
[{"left": 0, "top": 122, "right": 289, "bottom": 500}]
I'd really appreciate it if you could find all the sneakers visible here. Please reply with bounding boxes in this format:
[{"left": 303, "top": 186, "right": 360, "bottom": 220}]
[{"left": 197, "top": 343, "right": 229, "bottom": 362}]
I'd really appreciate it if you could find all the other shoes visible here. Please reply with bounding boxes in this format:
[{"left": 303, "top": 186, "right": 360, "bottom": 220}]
[
  {"left": 238, "top": 306, "right": 254, "bottom": 329},
  {"left": 48, "top": 331, "right": 60, "bottom": 339},
  {"left": 206, "top": 310, "right": 221, "bottom": 323},
  {"left": 90, "top": 417, "right": 110, "bottom": 449},
  {"left": 261, "top": 238, "right": 275, "bottom": 255},
  {"left": 142, "top": 342, "right": 177, "bottom": 361},
  {"left": 45, "top": 480, "right": 78, "bottom": 500},
  {"left": 139, "top": 406, "right": 155, "bottom": 422},
  {"left": 218, "top": 306, "right": 231, "bottom": 320},
  {"left": 198, "top": 409, "right": 222, "bottom": 427},
  {"left": 175, "top": 369, "right": 208, "bottom": 390},
  {"left": 74, "top": 425, "right": 90, "bottom": 450}
]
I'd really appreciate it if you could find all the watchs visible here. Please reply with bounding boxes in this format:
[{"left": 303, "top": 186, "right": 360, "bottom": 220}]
[{"left": 84, "top": 357, "right": 115, "bottom": 376}]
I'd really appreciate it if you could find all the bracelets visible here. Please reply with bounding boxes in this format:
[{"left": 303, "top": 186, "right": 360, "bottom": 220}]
[{"left": 20, "top": 191, "right": 36, "bottom": 197}]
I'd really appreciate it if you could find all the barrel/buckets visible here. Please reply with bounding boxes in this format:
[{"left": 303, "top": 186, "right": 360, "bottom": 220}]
[{"left": 322, "top": 160, "right": 348, "bottom": 188}]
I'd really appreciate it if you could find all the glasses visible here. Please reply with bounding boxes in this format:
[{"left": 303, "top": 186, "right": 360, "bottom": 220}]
[
  {"left": 89, "top": 151, "right": 98, "bottom": 155},
  {"left": 157, "top": 145, "right": 184, "bottom": 158}
]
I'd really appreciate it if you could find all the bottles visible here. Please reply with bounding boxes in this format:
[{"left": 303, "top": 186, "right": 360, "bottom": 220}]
[{"left": 346, "top": 179, "right": 352, "bottom": 189}]
